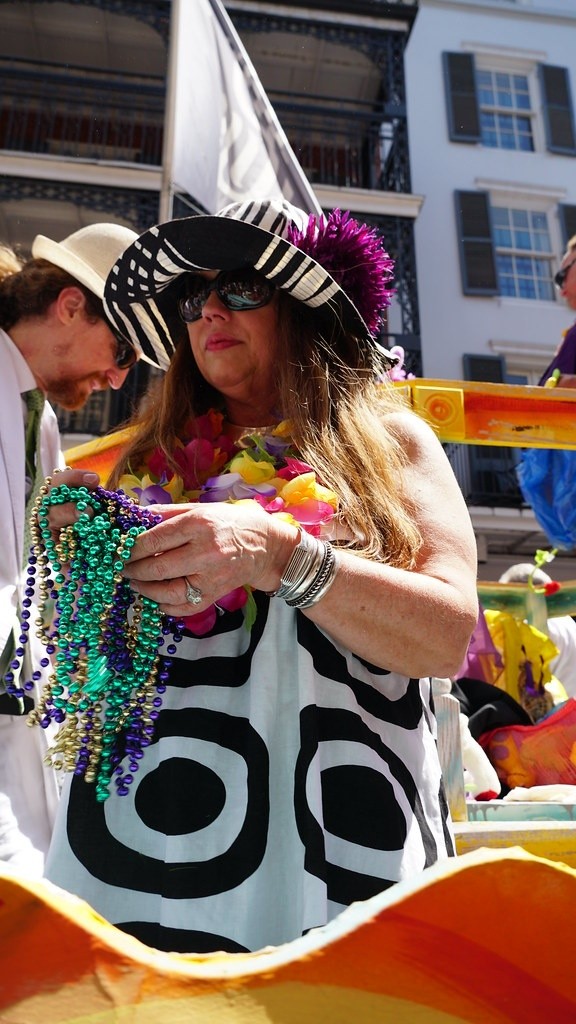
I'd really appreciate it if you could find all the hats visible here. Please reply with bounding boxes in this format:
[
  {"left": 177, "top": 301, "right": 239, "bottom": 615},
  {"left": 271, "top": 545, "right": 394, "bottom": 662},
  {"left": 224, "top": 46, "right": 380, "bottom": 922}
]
[
  {"left": 103, "top": 197, "right": 396, "bottom": 378},
  {"left": 33, "top": 222, "right": 159, "bottom": 367}
]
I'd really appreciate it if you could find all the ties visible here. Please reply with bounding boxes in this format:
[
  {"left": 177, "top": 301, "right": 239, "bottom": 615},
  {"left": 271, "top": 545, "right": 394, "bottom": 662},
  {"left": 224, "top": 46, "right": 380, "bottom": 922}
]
[{"left": 17, "top": 387, "right": 46, "bottom": 631}]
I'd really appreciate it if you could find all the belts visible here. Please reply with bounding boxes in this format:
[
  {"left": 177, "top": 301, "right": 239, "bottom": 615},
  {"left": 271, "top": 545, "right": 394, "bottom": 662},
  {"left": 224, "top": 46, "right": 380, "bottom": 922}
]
[{"left": 0, "top": 692, "right": 35, "bottom": 716}]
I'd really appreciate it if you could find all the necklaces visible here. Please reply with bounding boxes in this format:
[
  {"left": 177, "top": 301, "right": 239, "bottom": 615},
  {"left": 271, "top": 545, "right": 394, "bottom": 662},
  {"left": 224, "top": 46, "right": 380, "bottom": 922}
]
[
  {"left": 225, "top": 424, "right": 276, "bottom": 449},
  {"left": 3, "top": 466, "right": 185, "bottom": 799}
]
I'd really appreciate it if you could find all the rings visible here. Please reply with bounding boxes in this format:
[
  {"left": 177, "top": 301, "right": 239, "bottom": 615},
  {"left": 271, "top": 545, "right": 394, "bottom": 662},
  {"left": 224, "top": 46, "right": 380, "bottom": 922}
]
[{"left": 183, "top": 579, "right": 202, "bottom": 604}]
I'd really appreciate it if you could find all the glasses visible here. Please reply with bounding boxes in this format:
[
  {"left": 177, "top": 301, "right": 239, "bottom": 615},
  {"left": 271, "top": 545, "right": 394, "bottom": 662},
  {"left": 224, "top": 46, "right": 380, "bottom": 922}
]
[
  {"left": 176, "top": 269, "right": 275, "bottom": 322},
  {"left": 100, "top": 311, "right": 137, "bottom": 369},
  {"left": 554, "top": 259, "right": 576, "bottom": 285}
]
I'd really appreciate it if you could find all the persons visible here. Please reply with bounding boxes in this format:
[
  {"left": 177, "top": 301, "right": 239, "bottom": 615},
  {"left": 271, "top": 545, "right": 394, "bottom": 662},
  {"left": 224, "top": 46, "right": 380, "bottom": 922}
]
[
  {"left": 0, "top": 222, "right": 144, "bottom": 864},
  {"left": 28, "top": 198, "right": 480, "bottom": 957},
  {"left": 520, "top": 234, "right": 576, "bottom": 554},
  {"left": 495, "top": 562, "right": 576, "bottom": 700}
]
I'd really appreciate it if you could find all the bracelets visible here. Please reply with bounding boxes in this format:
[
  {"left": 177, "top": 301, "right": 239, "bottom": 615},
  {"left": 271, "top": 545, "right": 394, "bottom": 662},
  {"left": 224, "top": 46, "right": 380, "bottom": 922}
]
[{"left": 268, "top": 531, "right": 338, "bottom": 609}]
